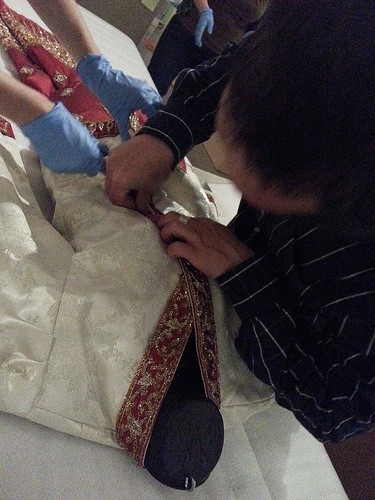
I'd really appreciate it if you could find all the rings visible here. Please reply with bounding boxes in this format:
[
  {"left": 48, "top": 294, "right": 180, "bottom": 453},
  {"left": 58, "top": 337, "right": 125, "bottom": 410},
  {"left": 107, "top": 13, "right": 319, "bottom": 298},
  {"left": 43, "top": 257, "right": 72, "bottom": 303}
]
[{"left": 178, "top": 214, "right": 190, "bottom": 225}]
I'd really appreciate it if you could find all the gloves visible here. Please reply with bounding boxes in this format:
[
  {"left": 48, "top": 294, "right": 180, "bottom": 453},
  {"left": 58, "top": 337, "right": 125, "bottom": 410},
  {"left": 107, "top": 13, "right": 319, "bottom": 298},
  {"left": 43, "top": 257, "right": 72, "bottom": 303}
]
[
  {"left": 195, "top": 8, "right": 214, "bottom": 47},
  {"left": 76, "top": 54, "right": 161, "bottom": 143},
  {"left": 19, "top": 101, "right": 109, "bottom": 177}
]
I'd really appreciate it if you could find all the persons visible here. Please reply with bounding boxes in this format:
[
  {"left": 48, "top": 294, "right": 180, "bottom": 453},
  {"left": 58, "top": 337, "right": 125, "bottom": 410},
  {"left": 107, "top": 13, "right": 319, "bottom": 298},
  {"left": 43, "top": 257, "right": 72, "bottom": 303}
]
[
  {"left": 0, "top": 0, "right": 164, "bottom": 177},
  {"left": 0, "top": 0, "right": 276, "bottom": 492},
  {"left": 148, "top": 0, "right": 272, "bottom": 98},
  {"left": 105, "top": 0, "right": 375, "bottom": 445}
]
